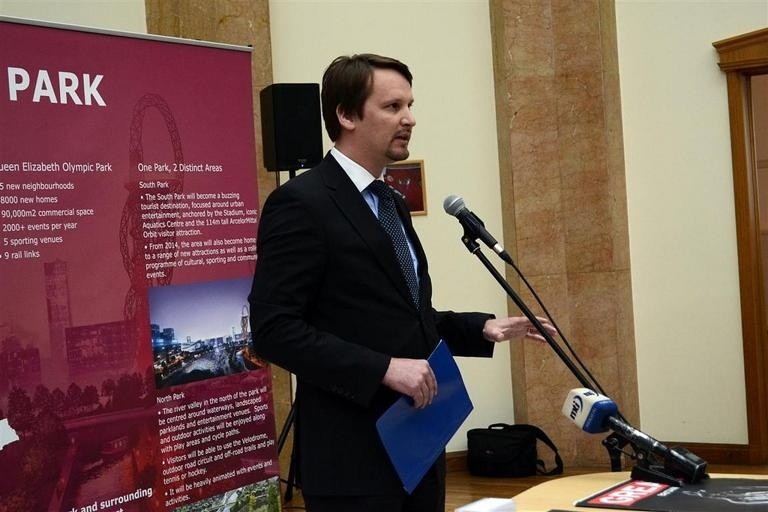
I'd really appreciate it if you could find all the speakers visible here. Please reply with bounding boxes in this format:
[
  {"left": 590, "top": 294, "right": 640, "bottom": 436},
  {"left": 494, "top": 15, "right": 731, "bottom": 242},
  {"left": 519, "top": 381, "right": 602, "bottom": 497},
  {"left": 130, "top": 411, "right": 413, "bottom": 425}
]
[{"left": 259, "top": 82, "right": 324, "bottom": 172}]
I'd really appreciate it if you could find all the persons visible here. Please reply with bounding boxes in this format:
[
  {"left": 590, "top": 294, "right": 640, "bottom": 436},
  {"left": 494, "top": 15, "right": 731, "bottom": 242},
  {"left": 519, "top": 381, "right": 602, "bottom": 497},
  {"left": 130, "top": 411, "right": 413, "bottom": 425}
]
[{"left": 247, "top": 52, "right": 557, "bottom": 511}]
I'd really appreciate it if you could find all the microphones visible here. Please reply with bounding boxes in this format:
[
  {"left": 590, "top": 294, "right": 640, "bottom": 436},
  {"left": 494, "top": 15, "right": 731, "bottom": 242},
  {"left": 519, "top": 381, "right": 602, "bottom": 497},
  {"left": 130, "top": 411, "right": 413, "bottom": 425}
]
[
  {"left": 561, "top": 388, "right": 707, "bottom": 484},
  {"left": 444, "top": 194, "right": 514, "bottom": 266}
]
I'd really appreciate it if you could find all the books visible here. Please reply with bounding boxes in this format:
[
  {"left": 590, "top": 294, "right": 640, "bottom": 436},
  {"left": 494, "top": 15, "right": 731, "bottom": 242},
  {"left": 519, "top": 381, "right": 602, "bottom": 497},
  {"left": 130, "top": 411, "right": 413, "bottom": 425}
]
[{"left": 375, "top": 338, "right": 475, "bottom": 496}]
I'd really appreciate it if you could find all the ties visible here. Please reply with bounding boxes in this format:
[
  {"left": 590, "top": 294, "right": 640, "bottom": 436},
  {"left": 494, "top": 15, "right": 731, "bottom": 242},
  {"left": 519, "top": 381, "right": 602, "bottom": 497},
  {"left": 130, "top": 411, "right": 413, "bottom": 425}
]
[{"left": 368, "top": 181, "right": 421, "bottom": 310}]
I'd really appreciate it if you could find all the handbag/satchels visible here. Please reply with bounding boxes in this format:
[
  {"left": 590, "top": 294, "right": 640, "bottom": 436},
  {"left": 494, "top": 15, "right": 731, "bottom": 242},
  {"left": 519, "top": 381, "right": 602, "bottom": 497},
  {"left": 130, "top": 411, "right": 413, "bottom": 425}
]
[{"left": 467, "top": 423, "right": 563, "bottom": 477}]
